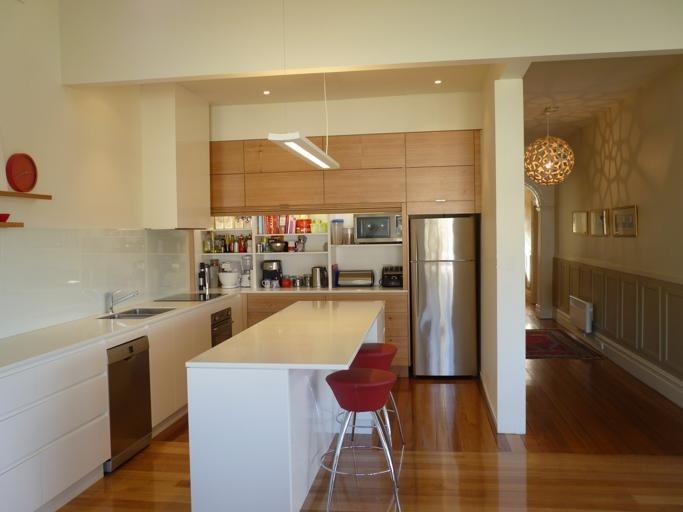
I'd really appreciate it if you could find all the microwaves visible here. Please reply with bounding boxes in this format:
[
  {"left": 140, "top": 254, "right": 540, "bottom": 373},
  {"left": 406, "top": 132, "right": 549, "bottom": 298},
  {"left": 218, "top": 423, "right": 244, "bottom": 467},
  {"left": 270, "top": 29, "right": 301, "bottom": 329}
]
[{"left": 353, "top": 212, "right": 402, "bottom": 245}]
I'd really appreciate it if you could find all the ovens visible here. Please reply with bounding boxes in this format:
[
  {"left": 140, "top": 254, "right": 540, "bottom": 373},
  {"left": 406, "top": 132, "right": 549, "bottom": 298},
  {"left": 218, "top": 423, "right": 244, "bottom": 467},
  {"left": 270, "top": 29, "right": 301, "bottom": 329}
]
[{"left": 209, "top": 307, "right": 232, "bottom": 347}]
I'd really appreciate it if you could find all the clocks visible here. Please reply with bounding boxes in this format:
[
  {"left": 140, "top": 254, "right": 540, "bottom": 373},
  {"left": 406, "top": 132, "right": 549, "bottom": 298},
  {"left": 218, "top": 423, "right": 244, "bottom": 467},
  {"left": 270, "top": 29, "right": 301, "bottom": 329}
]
[{"left": 5, "top": 152, "right": 38, "bottom": 193}]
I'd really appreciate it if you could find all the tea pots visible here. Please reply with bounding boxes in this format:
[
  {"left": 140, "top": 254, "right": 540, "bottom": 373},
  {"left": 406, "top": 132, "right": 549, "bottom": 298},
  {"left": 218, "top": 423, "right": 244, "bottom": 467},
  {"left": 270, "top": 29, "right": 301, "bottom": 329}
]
[{"left": 310, "top": 267, "right": 327, "bottom": 289}]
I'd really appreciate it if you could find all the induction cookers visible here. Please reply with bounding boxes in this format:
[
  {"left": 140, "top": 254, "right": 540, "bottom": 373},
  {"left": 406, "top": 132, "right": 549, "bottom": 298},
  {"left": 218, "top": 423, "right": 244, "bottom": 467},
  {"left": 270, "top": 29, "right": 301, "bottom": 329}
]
[{"left": 154, "top": 292, "right": 222, "bottom": 301}]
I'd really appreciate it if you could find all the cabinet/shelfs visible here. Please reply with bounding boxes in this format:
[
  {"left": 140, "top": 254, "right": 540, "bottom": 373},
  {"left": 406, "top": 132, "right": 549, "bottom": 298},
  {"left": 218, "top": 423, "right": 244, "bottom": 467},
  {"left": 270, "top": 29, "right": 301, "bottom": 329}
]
[
  {"left": 0, "top": 191, "right": 52, "bottom": 228},
  {"left": 1, "top": 344, "right": 112, "bottom": 512},
  {"left": 192, "top": 209, "right": 334, "bottom": 292},
  {"left": 325, "top": 294, "right": 410, "bottom": 367},
  {"left": 404, "top": 129, "right": 482, "bottom": 213},
  {"left": 209, "top": 141, "right": 246, "bottom": 216},
  {"left": 244, "top": 133, "right": 406, "bottom": 214}
]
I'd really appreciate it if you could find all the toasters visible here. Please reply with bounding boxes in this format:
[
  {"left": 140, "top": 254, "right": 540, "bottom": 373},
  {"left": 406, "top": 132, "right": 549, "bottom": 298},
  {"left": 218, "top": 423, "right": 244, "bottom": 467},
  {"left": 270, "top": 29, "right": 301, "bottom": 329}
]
[{"left": 381, "top": 265, "right": 403, "bottom": 287}]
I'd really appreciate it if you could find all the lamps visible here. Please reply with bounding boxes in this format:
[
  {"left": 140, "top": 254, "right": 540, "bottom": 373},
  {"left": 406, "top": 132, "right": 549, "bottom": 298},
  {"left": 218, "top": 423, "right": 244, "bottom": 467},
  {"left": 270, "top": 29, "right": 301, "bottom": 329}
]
[
  {"left": 267, "top": 70, "right": 341, "bottom": 170},
  {"left": 524, "top": 106, "right": 577, "bottom": 188}
]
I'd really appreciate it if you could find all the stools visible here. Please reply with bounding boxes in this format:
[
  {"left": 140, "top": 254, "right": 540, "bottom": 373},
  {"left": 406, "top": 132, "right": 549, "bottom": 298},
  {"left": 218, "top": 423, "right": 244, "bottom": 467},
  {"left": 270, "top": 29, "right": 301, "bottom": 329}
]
[{"left": 311, "top": 342, "right": 406, "bottom": 511}]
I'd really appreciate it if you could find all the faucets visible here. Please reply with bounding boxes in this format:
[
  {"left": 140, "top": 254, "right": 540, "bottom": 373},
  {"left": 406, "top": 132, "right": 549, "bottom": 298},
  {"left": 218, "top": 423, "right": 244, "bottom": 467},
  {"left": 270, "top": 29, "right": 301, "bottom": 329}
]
[{"left": 104, "top": 288, "right": 139, "bottom": 313}]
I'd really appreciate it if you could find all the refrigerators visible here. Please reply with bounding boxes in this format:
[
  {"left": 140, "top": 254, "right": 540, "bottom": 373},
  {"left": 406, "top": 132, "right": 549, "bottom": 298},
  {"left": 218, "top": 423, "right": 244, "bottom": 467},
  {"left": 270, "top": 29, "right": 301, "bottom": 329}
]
[{"left": 408, "top": 215, "right": 481, "bottom": 380}]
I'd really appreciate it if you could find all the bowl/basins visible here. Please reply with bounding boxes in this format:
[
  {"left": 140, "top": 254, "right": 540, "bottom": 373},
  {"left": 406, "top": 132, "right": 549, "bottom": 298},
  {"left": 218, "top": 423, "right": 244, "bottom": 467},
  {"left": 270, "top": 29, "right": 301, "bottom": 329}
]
[
  {"left": 217, "top": 273, "right": 239, "bottom": 287},
  {"left": 270, "top": 241, "right": 289, "bottom": 253}
]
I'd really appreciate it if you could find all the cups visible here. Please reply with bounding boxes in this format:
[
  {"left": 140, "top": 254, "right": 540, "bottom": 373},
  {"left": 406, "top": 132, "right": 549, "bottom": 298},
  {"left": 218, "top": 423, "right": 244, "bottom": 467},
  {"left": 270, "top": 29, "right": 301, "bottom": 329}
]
[
  {"left": 261, "top": 279, "right": 272, "bottom": 289},
  {"left": 342, "top": 227, "right": 354, "bottom": 246},
  {"left": 292, "top": 278, "right": 303, "bottom": 287}
]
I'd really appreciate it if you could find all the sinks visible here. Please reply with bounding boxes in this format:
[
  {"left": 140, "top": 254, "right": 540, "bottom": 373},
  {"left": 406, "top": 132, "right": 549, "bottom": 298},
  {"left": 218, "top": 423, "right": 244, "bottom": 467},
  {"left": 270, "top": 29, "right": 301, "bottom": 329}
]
[
  {"left": 95, "top": 313, "right": 153, "bottom": 324},
  {"left": 120, "top": 308, "right": 176, "bottom": 315}
]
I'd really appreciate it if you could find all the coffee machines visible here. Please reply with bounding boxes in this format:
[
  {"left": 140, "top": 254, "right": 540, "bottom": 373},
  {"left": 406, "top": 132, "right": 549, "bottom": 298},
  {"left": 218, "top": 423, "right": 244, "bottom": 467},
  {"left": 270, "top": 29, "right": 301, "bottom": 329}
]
[{"left": 261, "top": 260, "right": 281, "bottom": 289}]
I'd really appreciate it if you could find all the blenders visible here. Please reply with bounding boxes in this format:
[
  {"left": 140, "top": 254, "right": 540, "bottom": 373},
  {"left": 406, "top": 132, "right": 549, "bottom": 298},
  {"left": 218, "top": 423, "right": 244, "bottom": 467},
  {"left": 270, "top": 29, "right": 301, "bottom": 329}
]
[{"left": 239, "top": 255, "right": 252, "bottom": 288}]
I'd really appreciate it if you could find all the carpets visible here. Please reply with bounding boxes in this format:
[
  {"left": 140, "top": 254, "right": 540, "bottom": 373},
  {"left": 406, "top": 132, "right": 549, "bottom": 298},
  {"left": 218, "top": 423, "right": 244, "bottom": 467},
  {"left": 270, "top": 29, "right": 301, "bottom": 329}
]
[{"left": 526, "top": 328, "right": 603, "bottom": 359}]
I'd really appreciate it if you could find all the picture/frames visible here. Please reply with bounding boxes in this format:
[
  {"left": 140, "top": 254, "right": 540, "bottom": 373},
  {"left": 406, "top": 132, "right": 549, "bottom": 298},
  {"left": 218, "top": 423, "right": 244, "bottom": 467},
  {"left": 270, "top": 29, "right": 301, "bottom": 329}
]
[{"left": 570, "top": 202, "right": 638, "bottom": 237}]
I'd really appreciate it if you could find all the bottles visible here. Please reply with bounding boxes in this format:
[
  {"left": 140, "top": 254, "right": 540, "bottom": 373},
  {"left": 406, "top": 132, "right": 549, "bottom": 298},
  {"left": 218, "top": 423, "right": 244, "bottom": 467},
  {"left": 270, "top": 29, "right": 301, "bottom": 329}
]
[
  {"left": 280, "top": 274, "right": 291, "bottom": 288},
  {"left": 197, "top": 258, "right": 219, "bottom": 290},
  {"left": 331, "top": 220, "right": 345, "bottom": 246},
  {"left": 202, "top": 231, "right": 254, "bottom": 253}
]
